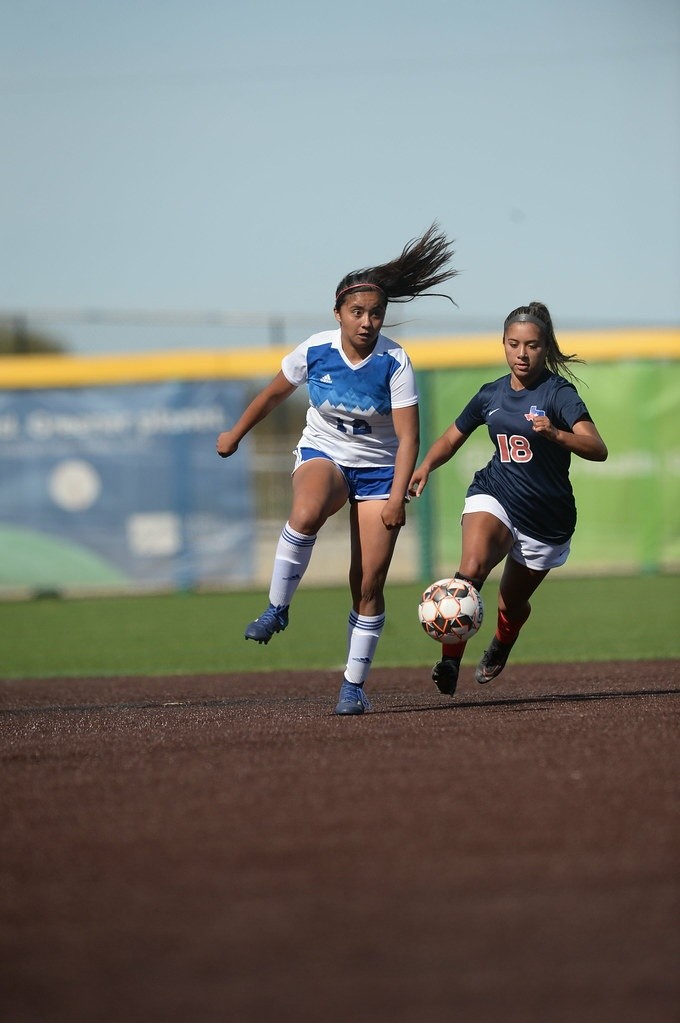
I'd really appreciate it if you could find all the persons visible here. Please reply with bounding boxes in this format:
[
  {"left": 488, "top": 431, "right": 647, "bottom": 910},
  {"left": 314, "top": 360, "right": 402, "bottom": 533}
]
[
  {"left": 408, "top": 302, "right": 608, "bottom": 696},
  {"left": 216, "top": 223, "right": 454, "bottom": 716}
]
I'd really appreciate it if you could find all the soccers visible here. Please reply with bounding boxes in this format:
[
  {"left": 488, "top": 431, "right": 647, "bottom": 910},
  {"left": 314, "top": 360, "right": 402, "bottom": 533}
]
[{"left": 417, "top": 577, "right": 484, "bottom": 645}]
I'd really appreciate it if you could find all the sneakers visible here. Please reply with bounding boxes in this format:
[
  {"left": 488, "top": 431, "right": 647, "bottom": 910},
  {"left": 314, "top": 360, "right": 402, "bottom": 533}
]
[
  {"left": 244, "top": 603, "right": 290, "bottom": 645},
  {"left": 475, "top": 633, "right": 519, "bottom": 684},
  {"left": 431, "top": 655, "right": 460, "bottom": 697},
  {"left": 336, "top": 677, "right": 372, "bottom": 714}
]
